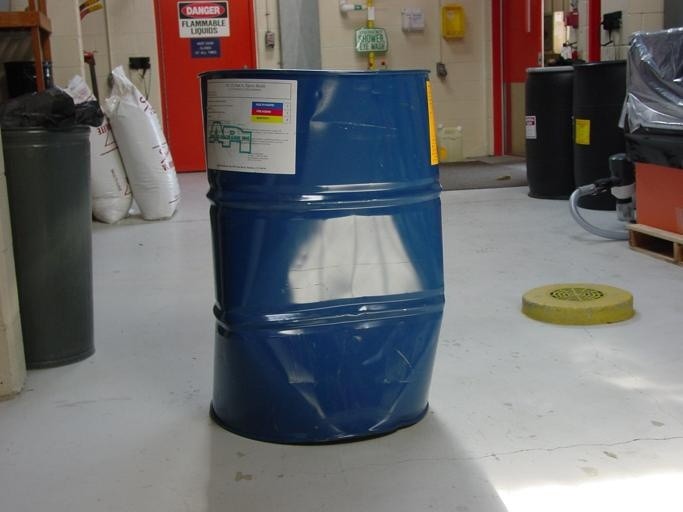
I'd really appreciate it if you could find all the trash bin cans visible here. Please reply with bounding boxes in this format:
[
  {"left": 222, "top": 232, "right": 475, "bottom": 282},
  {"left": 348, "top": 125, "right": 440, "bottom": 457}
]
[{"left": 1, "top": 126, "right": 95, "bottom": 370}]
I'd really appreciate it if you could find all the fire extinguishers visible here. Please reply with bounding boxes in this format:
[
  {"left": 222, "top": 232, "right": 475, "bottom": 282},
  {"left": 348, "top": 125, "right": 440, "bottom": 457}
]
[{"left": 571, "top": 4, "right": 578, "bottom": 28}]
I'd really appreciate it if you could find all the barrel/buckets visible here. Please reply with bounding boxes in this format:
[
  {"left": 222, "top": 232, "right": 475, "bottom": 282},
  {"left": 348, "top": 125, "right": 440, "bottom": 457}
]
[{"left": 197, "top": 65, "right": 451, "bottom": 447}]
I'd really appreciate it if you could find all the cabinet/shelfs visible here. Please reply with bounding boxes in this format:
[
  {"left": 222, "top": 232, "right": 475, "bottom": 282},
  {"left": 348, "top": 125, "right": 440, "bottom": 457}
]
[{"left": 0, "top": 0, "right": 52, "bottom": 94}]
